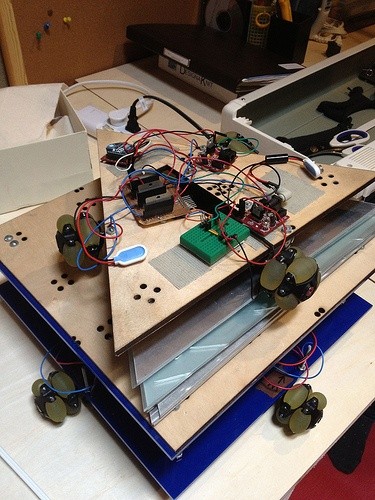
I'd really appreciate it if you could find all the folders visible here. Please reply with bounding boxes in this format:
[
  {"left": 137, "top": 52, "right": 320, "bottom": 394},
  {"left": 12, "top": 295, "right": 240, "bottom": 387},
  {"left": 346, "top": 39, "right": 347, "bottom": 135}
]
[{"left": 125, "top": 23, "right": 307, "bottom": 94}]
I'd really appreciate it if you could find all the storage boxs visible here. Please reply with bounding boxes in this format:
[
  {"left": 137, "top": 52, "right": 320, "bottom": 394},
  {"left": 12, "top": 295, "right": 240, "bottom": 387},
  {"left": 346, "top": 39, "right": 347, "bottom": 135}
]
[{"left": 0, "top": 88, "right": 95, "bottom": 212}]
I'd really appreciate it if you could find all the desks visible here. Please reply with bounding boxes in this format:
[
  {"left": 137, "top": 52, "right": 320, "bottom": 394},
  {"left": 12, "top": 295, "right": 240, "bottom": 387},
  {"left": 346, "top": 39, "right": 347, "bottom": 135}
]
[{"left": 1, "top": 64, "right": 375, "bottom": 500}]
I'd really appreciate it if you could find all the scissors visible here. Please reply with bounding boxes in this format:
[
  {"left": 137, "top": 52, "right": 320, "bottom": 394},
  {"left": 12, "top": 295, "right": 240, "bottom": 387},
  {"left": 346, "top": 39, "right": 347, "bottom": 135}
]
[{"left": 308, "top": 129, "right": 370, "bottom": 158}]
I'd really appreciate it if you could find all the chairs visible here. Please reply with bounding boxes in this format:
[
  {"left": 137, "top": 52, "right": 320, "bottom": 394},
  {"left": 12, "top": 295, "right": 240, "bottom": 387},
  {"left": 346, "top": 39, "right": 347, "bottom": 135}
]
[{"left": 1, "top": 0, "right": 199, "bottom": 97}]
